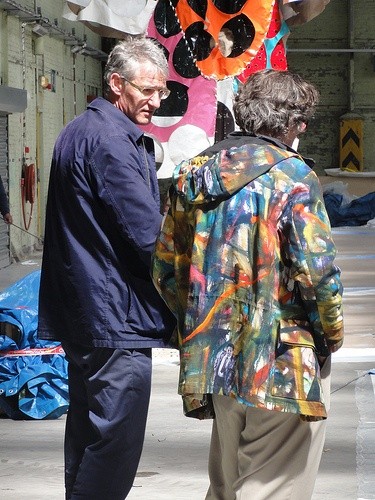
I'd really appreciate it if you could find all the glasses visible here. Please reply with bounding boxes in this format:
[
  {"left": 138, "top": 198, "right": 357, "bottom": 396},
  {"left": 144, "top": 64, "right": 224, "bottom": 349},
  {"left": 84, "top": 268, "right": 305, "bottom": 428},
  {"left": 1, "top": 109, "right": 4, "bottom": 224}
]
[{"left": 121, "top": 76, "right": 171, "bottom": 101}]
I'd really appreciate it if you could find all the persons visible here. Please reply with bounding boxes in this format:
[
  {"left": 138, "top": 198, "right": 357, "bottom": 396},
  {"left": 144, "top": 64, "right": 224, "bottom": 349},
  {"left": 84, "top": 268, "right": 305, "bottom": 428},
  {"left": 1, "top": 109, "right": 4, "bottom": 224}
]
[
  {"left": 0, "top": 175, "right": 12, "bottom": 224},
  {"left": 36, "top": 38, "right": 177, "bottom": 500},
  {"left": 151, "top": 68, "right": 344, "bottom": 500}
]
